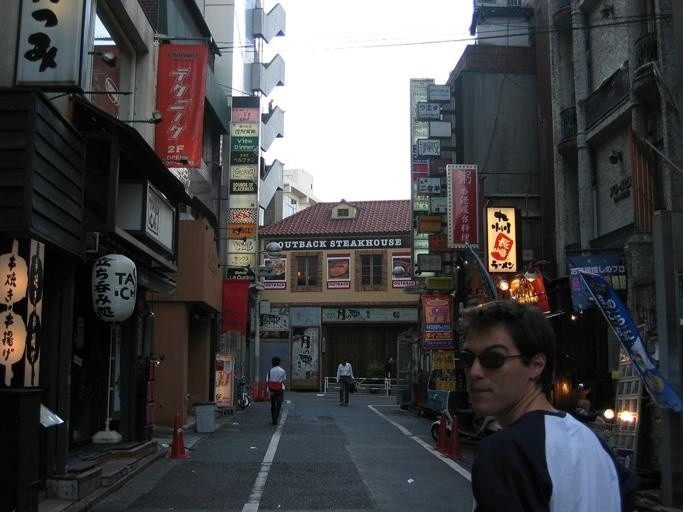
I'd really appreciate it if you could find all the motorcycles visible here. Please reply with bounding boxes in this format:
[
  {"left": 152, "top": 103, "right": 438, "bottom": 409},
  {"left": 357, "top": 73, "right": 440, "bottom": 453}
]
[{"left": 431, "top": 407, "right": 503, "bottom": 445}]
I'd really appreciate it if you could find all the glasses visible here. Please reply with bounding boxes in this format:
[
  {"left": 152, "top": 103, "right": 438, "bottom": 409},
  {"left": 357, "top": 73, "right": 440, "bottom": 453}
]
[{"left": 459, "top": 348, "right": 528, "bottom": 369}]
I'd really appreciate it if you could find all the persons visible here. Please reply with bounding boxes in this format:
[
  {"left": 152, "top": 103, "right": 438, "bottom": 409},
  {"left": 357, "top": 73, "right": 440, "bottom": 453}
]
[
  {"left": 458, "top": 300, "right": 635, "bottom": 512},
  {"left": 266, "top": 356, "right": 287, "bottom": 425},
  {"left": 384, "top": 355, "right": 394, "bottom": 396},
  {"left": 336, "top": 359, "right": 357, "bottom": 407}
]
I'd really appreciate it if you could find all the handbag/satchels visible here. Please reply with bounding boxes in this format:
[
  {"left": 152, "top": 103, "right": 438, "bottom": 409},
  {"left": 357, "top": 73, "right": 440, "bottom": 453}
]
[
  {"left": 268, "top": 382, "right": 282, "bottom": 392},
  {"left": 350, "top": 384, "right": 355, "bottom": 393}
]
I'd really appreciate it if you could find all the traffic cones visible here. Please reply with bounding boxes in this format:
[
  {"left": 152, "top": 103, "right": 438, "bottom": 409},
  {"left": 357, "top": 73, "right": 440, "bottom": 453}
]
[
  {"left": 432, "top": 413, "right": 465, "bottom": 461},
  {"left": 166, "top": 411, "right": 192, "bottom": 460}
]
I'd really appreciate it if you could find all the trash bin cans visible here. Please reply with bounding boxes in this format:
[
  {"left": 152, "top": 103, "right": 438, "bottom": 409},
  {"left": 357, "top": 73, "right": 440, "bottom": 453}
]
[{"left": 192, "top": 402, "right": 216, "bottom": 432}]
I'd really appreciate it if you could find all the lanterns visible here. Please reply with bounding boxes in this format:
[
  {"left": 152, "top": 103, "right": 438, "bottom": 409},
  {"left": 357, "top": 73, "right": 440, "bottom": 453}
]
[{"left": 91, "top": 253, "right": 137, "bottom": 322}]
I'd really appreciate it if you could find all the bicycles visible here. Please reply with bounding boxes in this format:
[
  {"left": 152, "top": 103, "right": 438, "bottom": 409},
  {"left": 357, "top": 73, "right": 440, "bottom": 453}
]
[{"left": 234, "top": 374, "right": 251, "bottom": 412}]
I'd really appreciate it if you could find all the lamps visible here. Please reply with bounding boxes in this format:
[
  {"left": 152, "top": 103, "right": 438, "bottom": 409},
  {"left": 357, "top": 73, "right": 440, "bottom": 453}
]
[
  {"left": 162, "top": 154, "right": 188, "bottom": 164},
  {"left": 122, "top": 110, "right": 163, "bottom": 125},
  {"left": 495, "top": 272, "right": 520, "bottom": 291},
  {"left": 523, "top": 260, "right": 554, "bottom": 283},
  {"left": 87, "top": 50, "right": 117, "bottom": 68},
  {"left": 391, "top": 265, "right": 412, "bottom": 278},
  {"left": 205, "top": 223, "right": 282, "bottom": 269}
]
